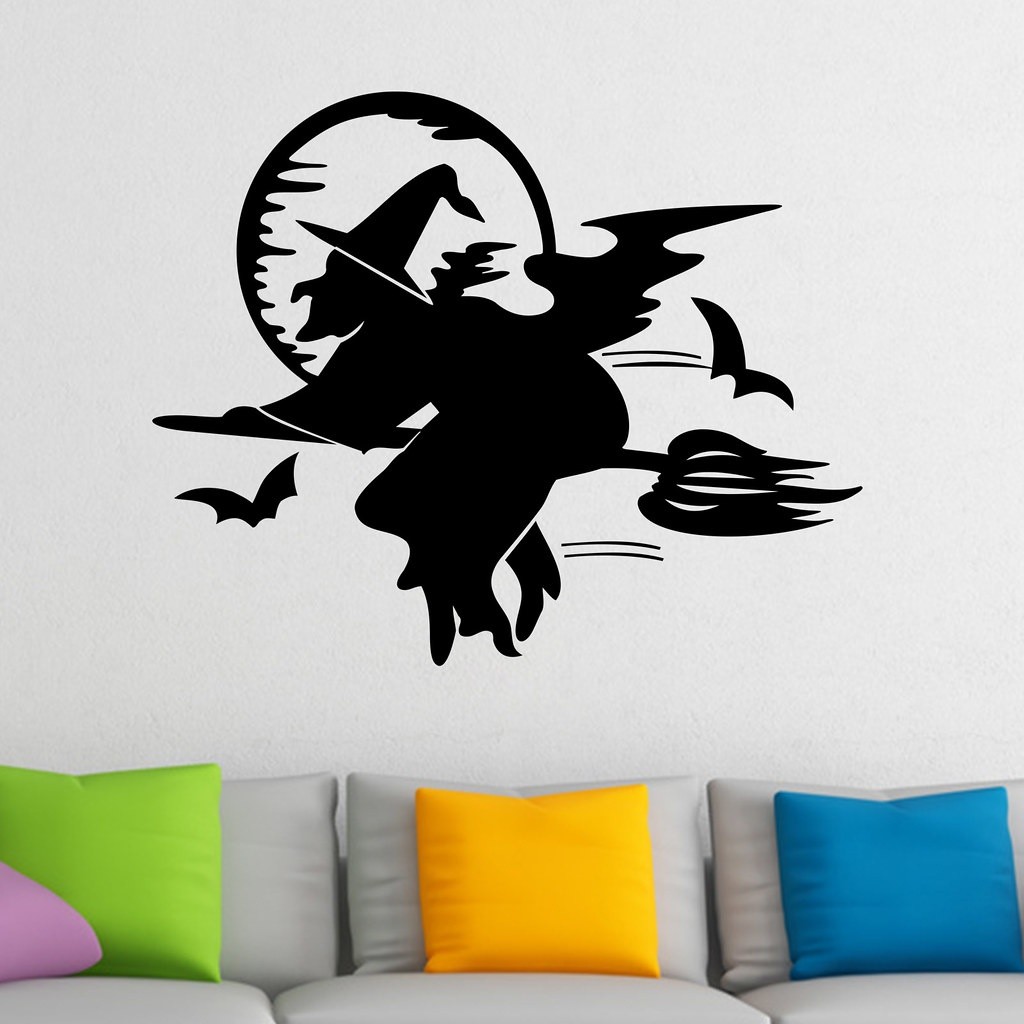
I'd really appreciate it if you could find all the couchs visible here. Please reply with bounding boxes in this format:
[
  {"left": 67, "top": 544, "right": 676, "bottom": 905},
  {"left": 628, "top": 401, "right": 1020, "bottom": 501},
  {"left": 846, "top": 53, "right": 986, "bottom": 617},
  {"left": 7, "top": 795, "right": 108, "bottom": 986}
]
[{"left": 0, "top": 775, "right": 1023, "bottom": 1023}]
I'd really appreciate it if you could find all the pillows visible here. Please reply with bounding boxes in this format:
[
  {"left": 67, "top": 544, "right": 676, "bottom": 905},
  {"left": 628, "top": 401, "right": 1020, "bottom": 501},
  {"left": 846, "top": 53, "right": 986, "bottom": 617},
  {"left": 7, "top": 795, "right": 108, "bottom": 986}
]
[
  {"left": 773, "top": 787, "right": 1023, "bottom": 980},
  {"left": 415, "top": 786, "right": 660, "bottom": 979},
  {"left": 0, "top": 764, "right": 221, "bottom": 983}
]
